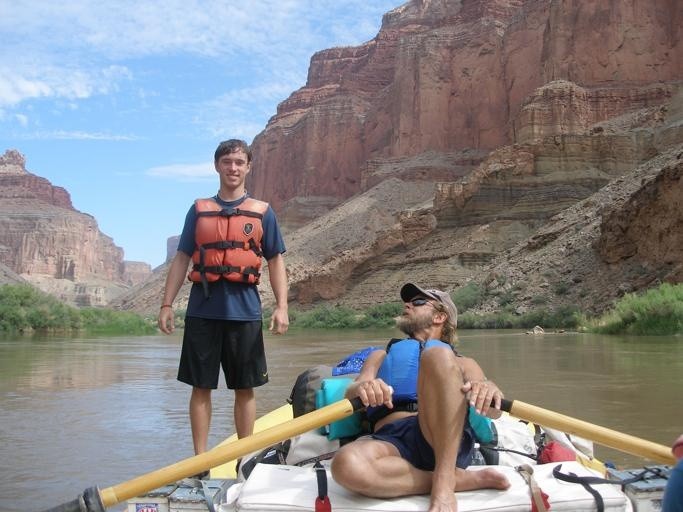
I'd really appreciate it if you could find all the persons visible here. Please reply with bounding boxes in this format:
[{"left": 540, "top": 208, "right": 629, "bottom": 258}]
[
  {"left": 153, "top": 137, "right": 290, "bottom": 480},
  {"left": 327, "top": 281, "right": 514, "bottom": 512}
]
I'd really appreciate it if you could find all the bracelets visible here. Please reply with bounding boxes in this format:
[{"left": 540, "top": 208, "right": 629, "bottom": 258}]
[{"left": 158, "top": 303, "right": 173, "bottom": 309}]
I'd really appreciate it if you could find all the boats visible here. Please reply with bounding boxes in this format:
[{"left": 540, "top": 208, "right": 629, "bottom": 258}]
[{"left": 116, "top": 396, "right": 638, "bottom": 512}]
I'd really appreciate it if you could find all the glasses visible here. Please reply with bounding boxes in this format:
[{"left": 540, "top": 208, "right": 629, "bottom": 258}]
[{"left": 405, "top": 296, "right": 444, "bottom": 314}]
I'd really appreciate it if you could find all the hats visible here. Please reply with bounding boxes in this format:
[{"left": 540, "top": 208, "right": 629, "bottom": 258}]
[{"left": 400, "top": 281, "right": 459, "bottom": 333}]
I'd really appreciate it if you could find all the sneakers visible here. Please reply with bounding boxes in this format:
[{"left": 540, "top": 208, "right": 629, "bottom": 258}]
[{"left": 190, "top": 470, "right": 210, "bottom": 480}]
